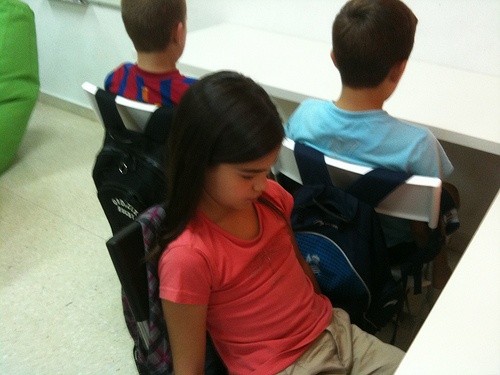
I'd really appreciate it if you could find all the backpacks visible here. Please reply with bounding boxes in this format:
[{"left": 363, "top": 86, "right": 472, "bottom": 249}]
[
  {"left": 117, "top": 205, "right": 173, "bottom": 375},
  {"left": 289, "top": 141, "right": 416, "bottom": 335},
  {"left": 93, "top": 89, "right": 175, "bottom": 237}
]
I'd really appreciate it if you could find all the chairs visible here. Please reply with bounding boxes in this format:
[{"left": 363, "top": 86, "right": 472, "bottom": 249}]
[
  {"left": 80, "top": 79, "right": 163, "bottom": 137},
  {"left": 275, "top": 137, "right": 454, "bottom": 346},
  {"left": 107, "top": 199, "right": 227, "bottom": 375}
]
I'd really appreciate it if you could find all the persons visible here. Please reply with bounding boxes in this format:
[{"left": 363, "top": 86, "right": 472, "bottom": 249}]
[
  {"left": 102, "top": 0, "right": 200, "bottom": 108},
  {"left": 157, "top": 69, "right": 406, "bottom": 375},
  {"left": 281, "top": 0, "right": 454, "bottom": 182}
]
